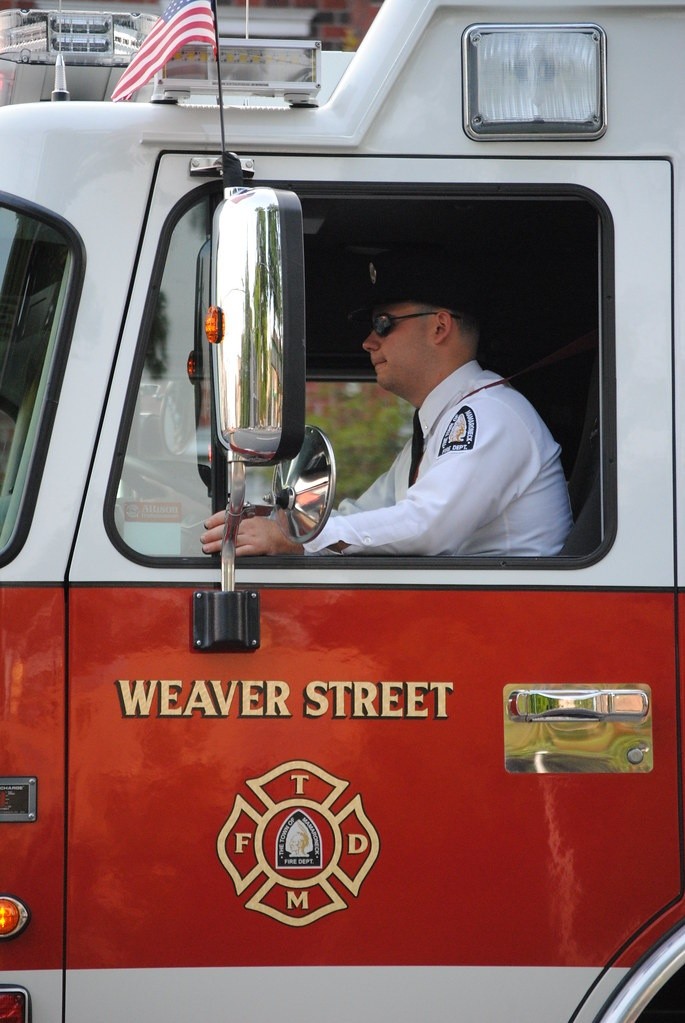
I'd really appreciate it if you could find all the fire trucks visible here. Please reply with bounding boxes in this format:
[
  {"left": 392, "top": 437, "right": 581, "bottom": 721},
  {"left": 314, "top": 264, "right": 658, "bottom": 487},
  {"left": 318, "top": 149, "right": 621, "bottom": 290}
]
[{"left": 1, "top": 10, "right": 684, "bottom": 1023}]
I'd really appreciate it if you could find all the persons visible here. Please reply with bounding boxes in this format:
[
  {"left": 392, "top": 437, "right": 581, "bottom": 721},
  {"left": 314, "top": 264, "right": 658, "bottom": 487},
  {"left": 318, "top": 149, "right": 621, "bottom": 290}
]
[{"left": 201, "top": 246, "right": 573, "bottom": 557}]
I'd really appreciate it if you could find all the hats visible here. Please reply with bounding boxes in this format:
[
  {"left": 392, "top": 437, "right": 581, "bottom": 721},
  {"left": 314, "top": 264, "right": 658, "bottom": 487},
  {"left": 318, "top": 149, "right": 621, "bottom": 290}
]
[{"left": 345, "top": 245, "right": 513, "bottom": 346}]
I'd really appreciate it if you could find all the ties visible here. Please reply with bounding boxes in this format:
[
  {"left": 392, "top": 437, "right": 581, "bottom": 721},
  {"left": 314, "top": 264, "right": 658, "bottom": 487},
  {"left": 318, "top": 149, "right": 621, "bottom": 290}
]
[{"left": 409, "top": 408, "right": 424, "bottom": 487}]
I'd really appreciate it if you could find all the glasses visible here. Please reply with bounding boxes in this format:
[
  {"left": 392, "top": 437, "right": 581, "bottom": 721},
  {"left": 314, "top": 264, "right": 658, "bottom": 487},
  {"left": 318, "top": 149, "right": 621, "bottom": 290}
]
[{"left": 368, "top": 312, "right": 460, "bottom": 338}]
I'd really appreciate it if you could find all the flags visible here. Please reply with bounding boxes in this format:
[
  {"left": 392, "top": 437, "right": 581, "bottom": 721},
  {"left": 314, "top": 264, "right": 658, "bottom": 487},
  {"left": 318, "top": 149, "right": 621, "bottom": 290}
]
[{"left": 111, "top": 0, "right": 217, "bottom": 103}]
